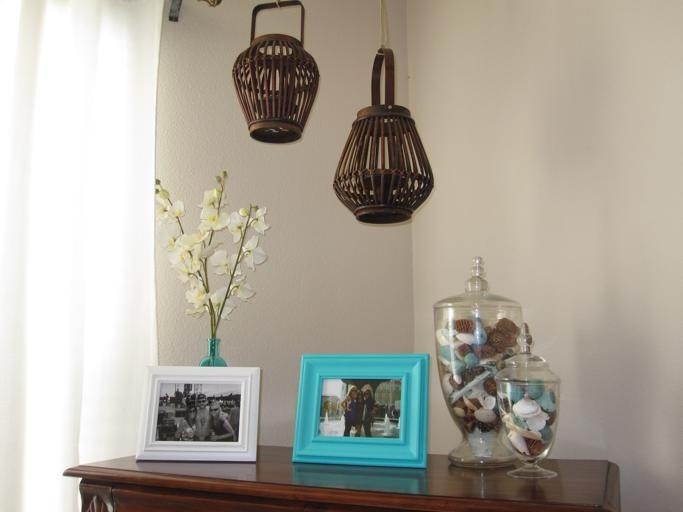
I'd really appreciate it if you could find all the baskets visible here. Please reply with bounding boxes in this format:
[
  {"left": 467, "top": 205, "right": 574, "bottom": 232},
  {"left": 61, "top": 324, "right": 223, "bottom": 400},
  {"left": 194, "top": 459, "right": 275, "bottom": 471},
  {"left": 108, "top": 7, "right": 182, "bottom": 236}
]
[
  {"left": 334, "top": 48, "right": 435, "bottom": 224},
  {"left": 233, "top": 0, "right": 320, "bottom": 144}
]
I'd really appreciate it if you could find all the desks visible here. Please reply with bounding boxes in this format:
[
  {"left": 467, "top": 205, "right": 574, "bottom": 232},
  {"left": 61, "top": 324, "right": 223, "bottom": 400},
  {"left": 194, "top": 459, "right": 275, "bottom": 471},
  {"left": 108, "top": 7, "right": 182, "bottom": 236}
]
[{"left": 62, "top": 440, "right": 623, "bottom": 512}]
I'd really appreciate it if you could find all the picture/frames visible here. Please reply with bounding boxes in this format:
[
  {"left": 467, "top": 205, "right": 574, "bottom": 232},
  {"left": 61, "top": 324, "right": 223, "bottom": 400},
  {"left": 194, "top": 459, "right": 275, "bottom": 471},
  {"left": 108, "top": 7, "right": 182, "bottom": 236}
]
[
  {"left": 290, "top": 353, "right": 431, "bottom": 469},
  {"left": 132, "top": 362, "right": 264, "bottom": 465}
]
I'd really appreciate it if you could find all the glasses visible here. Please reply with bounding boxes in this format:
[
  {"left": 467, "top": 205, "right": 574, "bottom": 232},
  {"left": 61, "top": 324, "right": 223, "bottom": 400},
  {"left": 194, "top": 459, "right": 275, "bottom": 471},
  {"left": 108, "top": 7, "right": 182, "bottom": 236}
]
[
  {"left": 209, "top": 407, "right": 219, "bottom": 412},
  {"left": 190, "top": 410, "right": 196, "bottom": 413},
  {"left": 197, "top": 400, "right": 206, "bottom": 404},
  {"left": 350, "top": 389, "right": 357, "bottom": 392},
  {"left": 363, "top": 391, "right": 370, "bottom": 395}
]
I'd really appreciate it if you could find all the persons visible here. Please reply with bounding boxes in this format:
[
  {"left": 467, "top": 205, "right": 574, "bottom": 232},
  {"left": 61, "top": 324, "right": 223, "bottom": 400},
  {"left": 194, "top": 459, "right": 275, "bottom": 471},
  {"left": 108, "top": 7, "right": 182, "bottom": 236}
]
[
  {"left": 229, "top": 401, "right": 240, "bottom": 442},
  {"left": 175, "top": 405, "right": 197, "bottom": 441},
  {"left": 195, "top": 394, "right": 211, "bottom": 441},
  {"left": 363, "top": 389, "right": 376, "bottom": 437},
  {"left": 355, "top": 390, "right": 364, "bottom": 437},
  {"left": 210, "top": 401, "right": 234, "bottom": 442},
  {"left": 341, "top": 386, "right": 356, "bottom": 436}
]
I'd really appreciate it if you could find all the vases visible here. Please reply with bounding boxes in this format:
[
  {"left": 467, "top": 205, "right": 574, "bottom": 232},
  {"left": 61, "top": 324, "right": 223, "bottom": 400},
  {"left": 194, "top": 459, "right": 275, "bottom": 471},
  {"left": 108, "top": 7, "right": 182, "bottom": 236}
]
[{"left": 195, "top": 340, "right": 227, "bottom": 368}]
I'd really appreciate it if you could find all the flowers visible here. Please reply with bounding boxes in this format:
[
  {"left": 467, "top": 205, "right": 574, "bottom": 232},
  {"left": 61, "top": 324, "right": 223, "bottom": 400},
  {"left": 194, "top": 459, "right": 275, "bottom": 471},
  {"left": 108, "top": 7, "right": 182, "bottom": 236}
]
[{"left": 156, "top": 168, "right": 270, "bottom": 340}]
